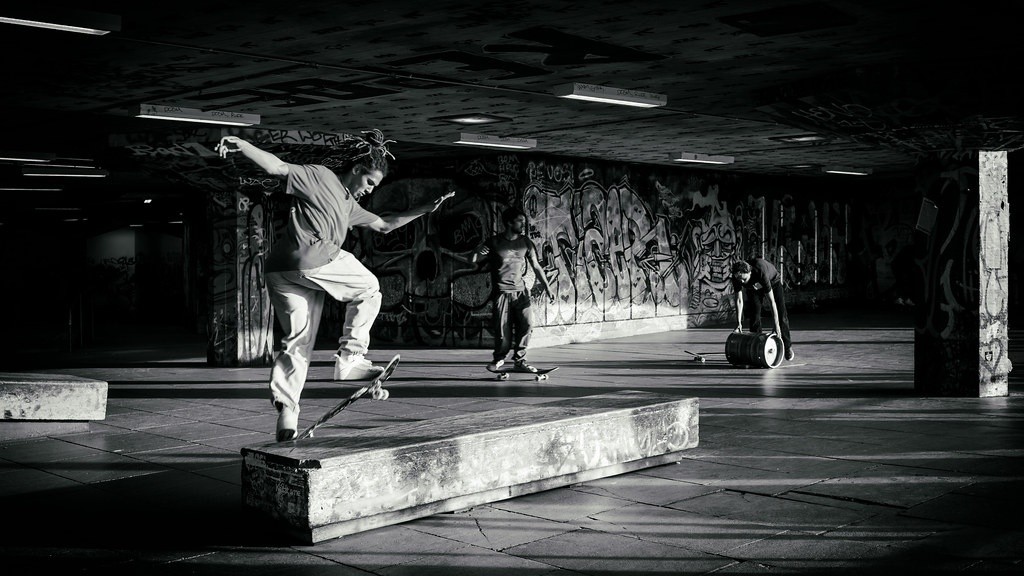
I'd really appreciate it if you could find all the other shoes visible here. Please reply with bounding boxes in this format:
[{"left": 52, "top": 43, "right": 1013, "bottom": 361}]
[
  {"left": 511, "top": 361, "right": 538, "bottom": 374},
  {"left": 786, "top": 347, "right": 795, "bottom": 360},
  {"left": 487, "top": 359, "right": 505, "bottom": 371}
]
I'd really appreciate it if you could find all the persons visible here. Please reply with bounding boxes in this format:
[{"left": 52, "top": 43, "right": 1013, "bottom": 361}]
[
  {"left": 214, "top": 129, "right": 455, "bottom": 442},
  {"left": 731, "top": 257, "right": 795, "bottom": 362},
  {"left": 469, "top": 207, "right": 556, "bottom": 373}
]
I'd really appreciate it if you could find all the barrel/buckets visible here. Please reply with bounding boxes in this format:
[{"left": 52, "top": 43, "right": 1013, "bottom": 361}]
[{"left": 724, "top": 331, "right": 785, "bottom": 369}]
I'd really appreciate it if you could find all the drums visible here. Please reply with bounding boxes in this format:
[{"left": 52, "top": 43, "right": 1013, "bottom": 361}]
[{"left": 725, "top": 331, "right": 785, "bottom": 369}]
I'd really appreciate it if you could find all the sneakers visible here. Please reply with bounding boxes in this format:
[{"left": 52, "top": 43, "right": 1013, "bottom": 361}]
[
  {"left": 333, "top": 354, "right": 386, "bottom": 381},
  {"left": 276, "top": 408, "right": 300, "bottom": 442}
]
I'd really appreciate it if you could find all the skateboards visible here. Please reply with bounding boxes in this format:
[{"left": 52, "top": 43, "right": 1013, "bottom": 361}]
[
  {"left": 296, "top": 353, "right": 401, "bottom": 439},
  {"left": 486, "top": 363, "right": 559, "bottom": 381}
]
